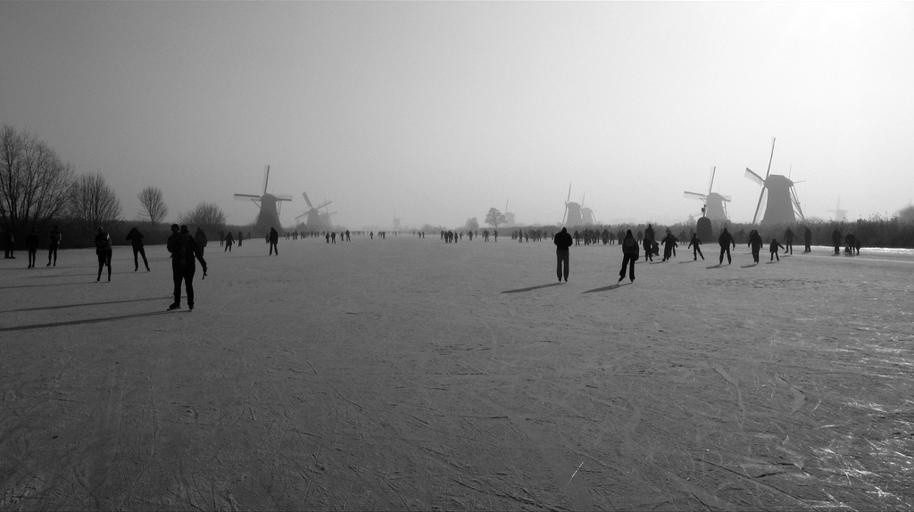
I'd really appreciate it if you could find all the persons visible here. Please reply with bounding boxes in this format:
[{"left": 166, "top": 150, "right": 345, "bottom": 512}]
[
  {"left": 719, "top": 228, "right": 735, "bottom": 264},
  {"left": 3, "top": 223, "right": 279, "bottom": 311},
  {"left": 831, "top": 227, "right": 841, "bottom": 254},
  {"left": 748, "top": 230, "right": 762, "bottom": 263},
  {"left": 784, "top": 228, "right": 798, "bottom": 255},
  {"left": 688, "top": 233, "right": 705, "bottom": 260},
  {"left": 803, "top": 225, "right": 812, "bottom": 251},
  {"left": 441, "top": 223, "right": 678, "bottom": 284},
  {"left": 768, "top": 238, "right": 785, "bottom": 262},
  {"left": 285, "top": 228, "right": 426, "bottom": 243},
  {"left": 844, "top": 233, "right": 862, "bottom": 256}
]
